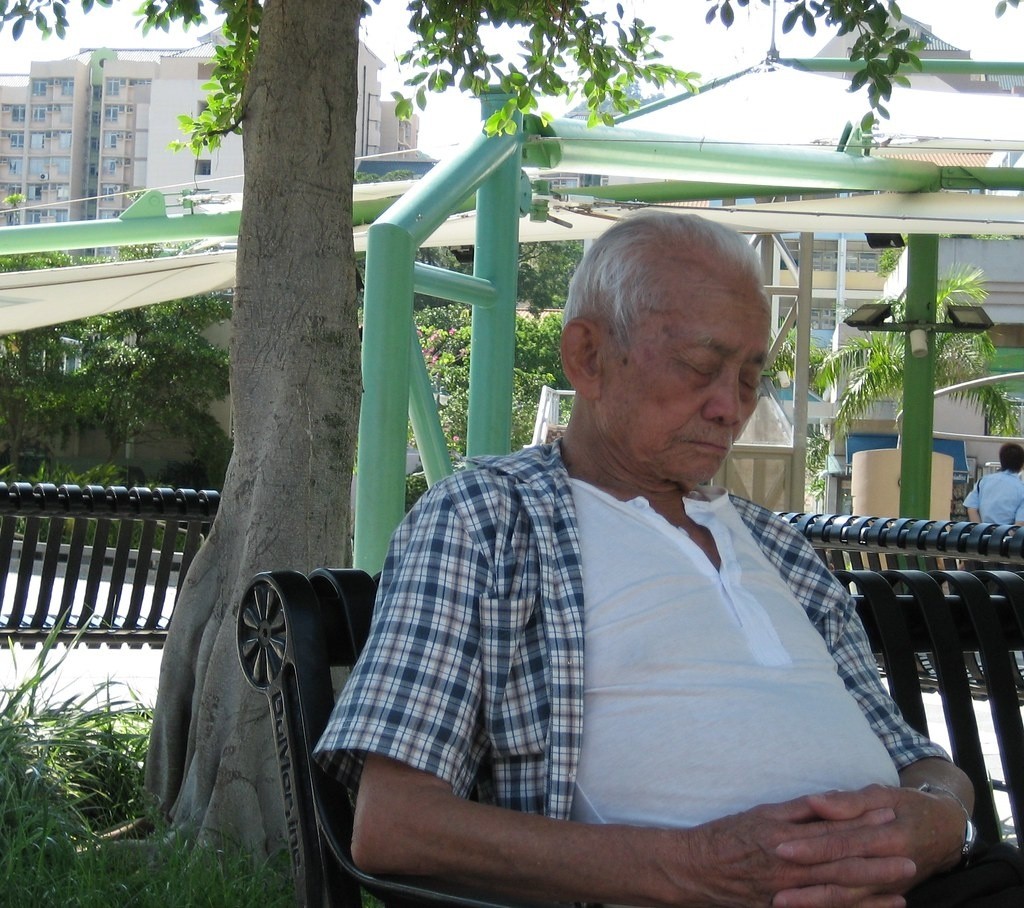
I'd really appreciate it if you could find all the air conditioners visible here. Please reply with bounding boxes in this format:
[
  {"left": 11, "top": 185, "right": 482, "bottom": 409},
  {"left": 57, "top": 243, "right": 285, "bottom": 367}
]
[
  {"left": 113, "top": 186, "right": 121, "bottom": 192},
  {"left": 40, "top": 173, "right": 48, "bottom": 180}
]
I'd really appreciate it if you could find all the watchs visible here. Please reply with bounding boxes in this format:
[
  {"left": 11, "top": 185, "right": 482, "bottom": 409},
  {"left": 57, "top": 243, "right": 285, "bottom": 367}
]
[{"left": 916, "top": 781, "right": 979, "bottom": 857}]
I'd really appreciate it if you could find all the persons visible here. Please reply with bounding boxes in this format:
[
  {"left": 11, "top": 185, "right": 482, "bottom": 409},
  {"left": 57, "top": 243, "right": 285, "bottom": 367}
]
[
  {"left": 313, "top": 207, "right": 1024, "bottom": 908},
  {"left": 958, "top": 443, "right": 1024, "bottom": 570}
]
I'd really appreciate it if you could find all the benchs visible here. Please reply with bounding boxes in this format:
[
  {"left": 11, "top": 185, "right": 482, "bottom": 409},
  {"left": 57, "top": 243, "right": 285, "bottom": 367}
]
[
  {"left": 0, "top": 481, "right": 221, "bottom": 649},
  {"left": 238, "top": 510, "right": 1024, "bottom": 908}
]
